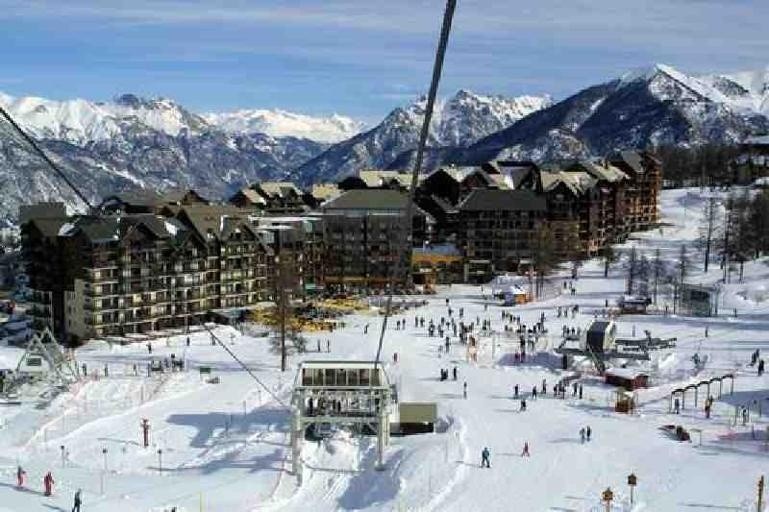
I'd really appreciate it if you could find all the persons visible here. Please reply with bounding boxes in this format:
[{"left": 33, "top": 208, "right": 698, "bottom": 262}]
[
  {"left": 17, "top": 464, "right": 26, "bottom": 488},
  {"left": 392, "top": 281, "right": 769, "bottom": 471},
  {"left": 71, "top": 486, "right": 84, "bottom": 512},
  {"left": 45, "top": 472, "right": 55, "bottom": 494}
]
